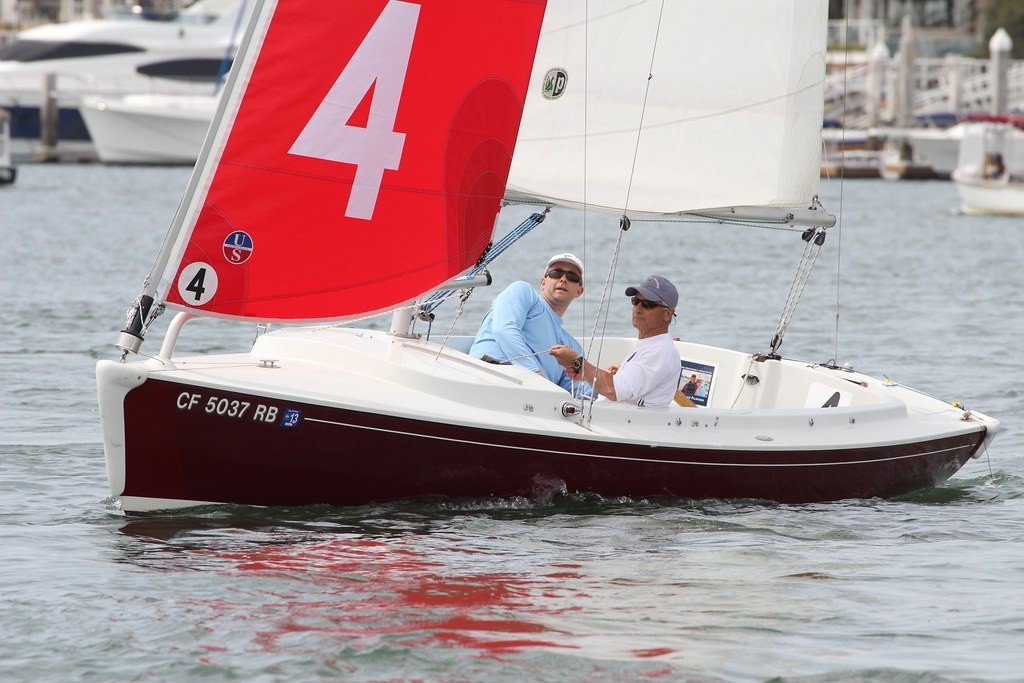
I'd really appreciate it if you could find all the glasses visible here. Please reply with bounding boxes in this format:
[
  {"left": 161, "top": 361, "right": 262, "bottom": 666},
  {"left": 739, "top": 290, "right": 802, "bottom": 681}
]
[
  {"left": 545, "top": 268, "right": 581, "bottom": 287},
  {"left": 630, "top": 297, "right": 677, "bottom": 317}
]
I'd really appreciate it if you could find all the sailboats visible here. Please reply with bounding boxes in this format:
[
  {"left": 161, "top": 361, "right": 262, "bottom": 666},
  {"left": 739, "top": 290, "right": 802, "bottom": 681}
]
[{"left": 91, "top": 0, "right": 1002, "bottom": 518}]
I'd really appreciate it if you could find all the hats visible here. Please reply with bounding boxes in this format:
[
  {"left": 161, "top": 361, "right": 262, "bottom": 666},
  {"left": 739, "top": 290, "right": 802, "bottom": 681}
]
[
  {"left": 544, "top": 253, "right": 584, "bottom": 286},
  {"left": 625, "top": 276, "right": 679, "bottom": 311}
]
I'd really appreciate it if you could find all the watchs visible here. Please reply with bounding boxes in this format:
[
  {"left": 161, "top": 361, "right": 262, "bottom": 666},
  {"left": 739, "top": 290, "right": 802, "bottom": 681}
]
[{"left": 571, "top": 357, "right": 583, "bottom": 374}]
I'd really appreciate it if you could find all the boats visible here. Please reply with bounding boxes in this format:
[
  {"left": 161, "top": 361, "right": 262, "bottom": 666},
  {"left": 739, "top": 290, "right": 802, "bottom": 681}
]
[
  {"left": 0, "top": 0, "right": 248, "bottom": 166},
  {"left": 78, "top": 91, "right": 217, "bottom": 166},
  {"left": 948, "top": 115, "right": 1024, "bottom": 220},
  {"left": 819, "top": 125, "right": 940, "bottom": 181}
]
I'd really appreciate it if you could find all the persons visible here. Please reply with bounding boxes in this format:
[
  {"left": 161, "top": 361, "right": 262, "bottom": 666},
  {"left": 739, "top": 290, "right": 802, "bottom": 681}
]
[
  {"left": 551, "top": 276, "right": 681, "bottom": 410},
  {"left": 682, "top": 375, "right": 704, "bottom": 399},
  {"left": 468, "top": 251, "right": 623, "bottom": 402}
]
